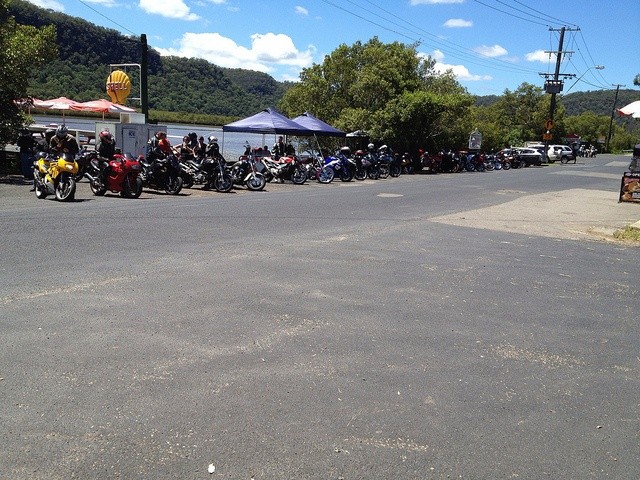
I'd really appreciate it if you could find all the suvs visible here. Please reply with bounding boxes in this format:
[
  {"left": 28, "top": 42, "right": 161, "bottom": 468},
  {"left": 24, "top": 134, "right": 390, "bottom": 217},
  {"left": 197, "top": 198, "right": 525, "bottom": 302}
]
[
  {"left": 548, "top": 145, "right": 573, "bottom": 164},
  {"left": 519, "top": 148, "right": 546, "bottom": 163}
]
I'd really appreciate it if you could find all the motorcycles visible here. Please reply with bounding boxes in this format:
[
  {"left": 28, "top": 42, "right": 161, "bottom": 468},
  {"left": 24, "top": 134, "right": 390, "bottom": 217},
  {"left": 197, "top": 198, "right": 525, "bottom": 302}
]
[
  {"left": 135, "top": 140, "right": 182, "bottom": 195},
  {"left": 260, "top": 153, "right": 307, "bottom": 185},
  {"left": 299, "top": 150, "right": 335, "bottom": 184},
  {"left": 75, "top": 149, "right": 95, "bottom": 182},
  {"left": 31, "top": 152, "right": 80, "bottom": 202},
  {"left": 179, "top": 144, "right": 233, "bottom": 193},
  {"left": 223, "top": 141, "right": 266, "bottom": 191},
  {"left": 322, "top": 142, "right": 523, "bottom": 182},
  {"left": 84, "top": 152, "right": 145, "bottom": 198}
]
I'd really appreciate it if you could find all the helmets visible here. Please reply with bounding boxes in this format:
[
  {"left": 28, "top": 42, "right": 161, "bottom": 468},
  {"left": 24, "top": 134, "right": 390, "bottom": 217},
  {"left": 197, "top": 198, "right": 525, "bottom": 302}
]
[
  {"left": 55, "top": 124, "right": 68, "bottom": 139},
  {"left": 45, "top": 129, "right": 55, "bottom": 141},
  {"left": 99, "top": 130, "right": 112, "bottom": 144}
]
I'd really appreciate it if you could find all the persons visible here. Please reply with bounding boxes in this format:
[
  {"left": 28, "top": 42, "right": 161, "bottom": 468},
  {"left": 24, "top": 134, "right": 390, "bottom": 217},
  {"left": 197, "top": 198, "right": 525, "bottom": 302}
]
[
  {"left": 48, "top": 125, "right": 79, "bottom": 200},
  {"left": 262, "top": 146, "right": 270, "bottom": 154},
  {"left": 35, "top": 127, "right": 56, "bottom": 195},
  {"left": 285, "top": 145, "right": 295, "bottom": 155},
  {"left": 95, "top": 131, "right": 122, "bottom": 187},
  {"left": 172, "top": 135, "right": 193, "bottom": 163},
  {"left": 193, "top": 136, "right": 207, "bottom": 161},
  {"left": 271, "top": 136, "right": 284, "bottom": 183},
  {"left": 158, "top": 131, "right": 172, "bottom": 155},
  {"left": 201, "top": 136, "right": 219, "bottom": 190},
  {"left": 188, "top": 132, "right": 199, "bottom": 162},
  {"left": 571, "top": 146, "right": 577, "bottom": 164},
  {"left": 244, "top": 145, "right": 252, "bottom": 155},
  {"left": 17, "top": 127, "right": 35, "bottom": 184},
  {"left": 146, "top": 131, "right": 166, "bottom": 164},
  {"left": 577, "top": 144, "right": 596, "bottom": 158}
]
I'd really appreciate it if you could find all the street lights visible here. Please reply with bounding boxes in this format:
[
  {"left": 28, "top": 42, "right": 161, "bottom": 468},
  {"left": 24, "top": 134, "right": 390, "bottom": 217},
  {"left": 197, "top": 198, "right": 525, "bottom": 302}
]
[{"left": 556, "top": 64, "right": 604, "bottom": 102}]
